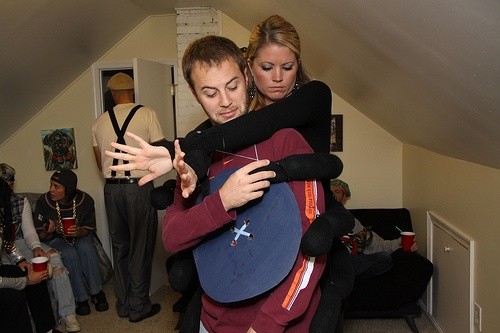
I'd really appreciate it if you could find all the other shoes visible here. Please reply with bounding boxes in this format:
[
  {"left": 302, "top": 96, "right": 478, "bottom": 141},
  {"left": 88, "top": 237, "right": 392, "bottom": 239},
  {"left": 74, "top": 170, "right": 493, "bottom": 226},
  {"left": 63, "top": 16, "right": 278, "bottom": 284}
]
[
  {"left": 129, "top": 303, "right": 161, "bottom": 322},
  {"left": 51, "top": 329, "right": 62, "bottom": 333},
  {"left": 75, "top": 299, "right": 90, "bottom": 316},
  {"left": 92, "top": 289, "right": 109, "bottom": 312}
]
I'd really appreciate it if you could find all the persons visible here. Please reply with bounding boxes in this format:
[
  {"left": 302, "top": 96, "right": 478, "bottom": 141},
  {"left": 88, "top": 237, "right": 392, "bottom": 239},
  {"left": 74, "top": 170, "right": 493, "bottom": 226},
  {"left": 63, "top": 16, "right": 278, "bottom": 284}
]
[
  {"left": 104, "top": 13, "right": 354, "bottom": 333},
  {"left": 0, "top": 163, "right": 81, "bottom": 333},
  {"left": 92, "top": 72, "right": 167, "bottom": 322},
  {"left": 329, "top": 179, "right": 418, "bottom": 277},
  {"left": 34, "top": 168, "right": 109, "bottom": 316}
]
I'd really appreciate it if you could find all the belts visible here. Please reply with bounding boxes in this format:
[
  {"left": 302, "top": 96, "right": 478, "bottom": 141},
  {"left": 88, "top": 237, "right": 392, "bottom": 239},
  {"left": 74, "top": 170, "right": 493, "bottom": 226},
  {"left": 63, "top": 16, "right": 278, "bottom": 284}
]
[{"left": 105, "top": 178, "right": 153, "bottom": 184}]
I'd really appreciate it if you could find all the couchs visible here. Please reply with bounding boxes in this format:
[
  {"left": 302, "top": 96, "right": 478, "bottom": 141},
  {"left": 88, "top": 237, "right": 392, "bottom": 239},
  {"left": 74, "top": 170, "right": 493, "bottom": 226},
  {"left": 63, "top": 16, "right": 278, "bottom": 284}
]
[
  {"left": 347, "top": 208, "right": 434, "bottom": 320},
  {"left": 17, "top": 191, "right": 113, "bottom": 333}
]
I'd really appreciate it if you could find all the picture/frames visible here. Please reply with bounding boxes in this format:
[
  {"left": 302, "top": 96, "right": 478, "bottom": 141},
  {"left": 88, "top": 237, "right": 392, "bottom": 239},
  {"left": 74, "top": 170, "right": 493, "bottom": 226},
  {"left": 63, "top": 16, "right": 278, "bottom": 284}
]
[{"left": 331, "top": 114, "right": 344, "bottom": 151}]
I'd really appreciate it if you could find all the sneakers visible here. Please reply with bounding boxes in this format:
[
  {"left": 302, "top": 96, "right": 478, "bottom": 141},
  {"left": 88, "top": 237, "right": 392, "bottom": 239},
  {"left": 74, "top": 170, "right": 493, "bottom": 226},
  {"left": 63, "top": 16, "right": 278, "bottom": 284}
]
[{"left": 60, "top": 313, "right": 81, "bottom": 332}]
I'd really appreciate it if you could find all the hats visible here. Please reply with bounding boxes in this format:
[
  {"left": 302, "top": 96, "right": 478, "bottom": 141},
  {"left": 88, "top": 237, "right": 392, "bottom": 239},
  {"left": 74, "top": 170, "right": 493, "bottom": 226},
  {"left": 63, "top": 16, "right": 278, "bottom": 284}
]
[
  {"left": 107, "top": 72, "right": 134, "bottom": 90},
  {"left": 51, "top": 168, "right": 77, "bottom": 189}
]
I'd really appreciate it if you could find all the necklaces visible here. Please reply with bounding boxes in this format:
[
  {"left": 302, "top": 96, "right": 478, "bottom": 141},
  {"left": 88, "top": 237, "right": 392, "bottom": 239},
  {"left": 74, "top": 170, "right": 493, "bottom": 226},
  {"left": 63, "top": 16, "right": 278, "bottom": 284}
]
[
  {"left": 56, "top": 199, "right": 76, "bottom": 245},
  {"left": 213, "top": 144, "right": 258, "bottom": 161}
]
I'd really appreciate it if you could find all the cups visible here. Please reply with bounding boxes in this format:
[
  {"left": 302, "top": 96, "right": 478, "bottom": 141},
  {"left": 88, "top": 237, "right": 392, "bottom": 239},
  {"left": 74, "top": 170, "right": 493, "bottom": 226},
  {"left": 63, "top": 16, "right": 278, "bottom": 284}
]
[
  {"left": 400, "top": 232, "right": 416, "bottom": 252},
  {"left": 30, "top": 257, "right": 49, "bottom": 272},
  {"left": 62, "top": 217, "right": 76, "bottom": 235}
]
